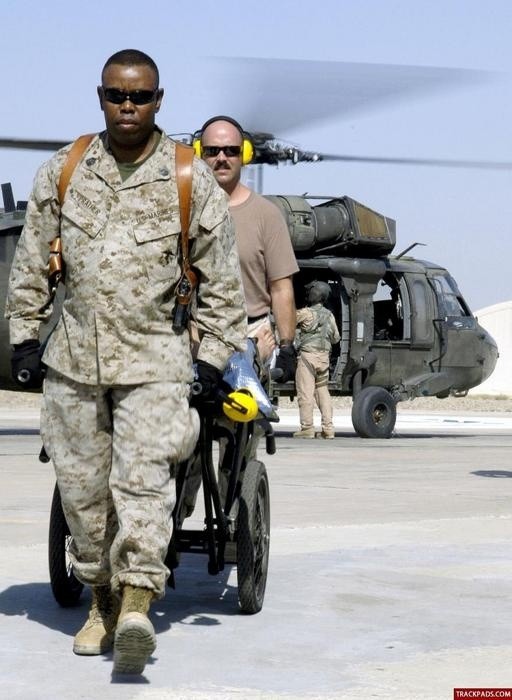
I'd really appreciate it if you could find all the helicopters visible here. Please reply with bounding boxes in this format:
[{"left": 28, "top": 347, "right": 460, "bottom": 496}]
[{"left": 1, "top": 54, "right": 511, "bottom": 437}]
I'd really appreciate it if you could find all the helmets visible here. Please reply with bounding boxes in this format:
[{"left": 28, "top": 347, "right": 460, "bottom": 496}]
[{"left": 303, "top": 281, "right": 332, "bottom": 302}]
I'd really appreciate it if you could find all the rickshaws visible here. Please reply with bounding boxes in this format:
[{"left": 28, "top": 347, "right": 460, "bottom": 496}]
[{"left": 12, "top": 363, "right": 290, "bottom": 617}]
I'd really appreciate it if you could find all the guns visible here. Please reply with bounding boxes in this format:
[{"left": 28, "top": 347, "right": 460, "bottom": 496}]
[{"left": 173, "top": 277, "right": 192, "bottom": 336}]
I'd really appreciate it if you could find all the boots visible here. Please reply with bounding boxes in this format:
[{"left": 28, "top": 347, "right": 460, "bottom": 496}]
[
  {"left": 113, "top": 584, "right": 157, "bottom": 674},
  {"left": 73, "top": 585, "right": 120, "bottom": 655}
]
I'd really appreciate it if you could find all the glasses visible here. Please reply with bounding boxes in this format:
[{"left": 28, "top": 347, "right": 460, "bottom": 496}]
[
  {"left": 200, "top": 146, "right": 240, "bottom": 158},
  {"left": 103, "top": 89, "right": 155, "bottom": 104}
]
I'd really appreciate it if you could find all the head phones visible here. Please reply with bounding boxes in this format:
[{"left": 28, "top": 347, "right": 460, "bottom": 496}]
[
  {"left": 217, "top": 387, "right": 257, "bottom": 423},
  {"left": 191, "top": 116, "right": 256, "bottom": 166}
]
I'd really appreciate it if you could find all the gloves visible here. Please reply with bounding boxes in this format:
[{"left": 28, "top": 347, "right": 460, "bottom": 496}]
[
  {"left": 276, "top": 345, "right": 296, "bottom": 382},
  {"left": 196, "top": 366, "right": 217, "bottom": 396},
  {"left": 11, "top": 339, "right": 41, "bottom": 389}
]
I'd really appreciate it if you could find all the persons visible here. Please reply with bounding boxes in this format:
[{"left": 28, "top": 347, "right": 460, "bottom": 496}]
[
  {"left": 289, "top": 278, "right": 344, "bottom": 439},
  {"left": 192, "top": 116, "right": 296, "bottom": 540},
  {"left": 7, "top": 48, "right": 250, "bottom": 680}
]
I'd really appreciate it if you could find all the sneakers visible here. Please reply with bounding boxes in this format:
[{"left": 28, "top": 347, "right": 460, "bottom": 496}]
[
  {"left": 322, "top": 432, "right": 335, "bottom": 439},
  {"left": 294, "top": 431, "right": 316, "bottom": 438}
]
